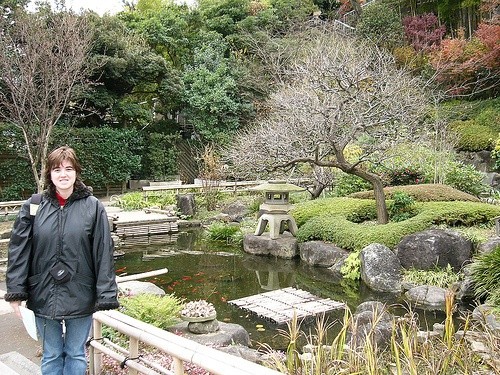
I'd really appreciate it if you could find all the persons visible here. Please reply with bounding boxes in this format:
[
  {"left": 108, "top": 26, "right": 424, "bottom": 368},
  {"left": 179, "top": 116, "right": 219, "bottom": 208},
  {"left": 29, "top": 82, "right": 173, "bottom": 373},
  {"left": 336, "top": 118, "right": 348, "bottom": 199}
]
[{"left": 4, "top": 146, "right": 119, "bottom": 375}]
